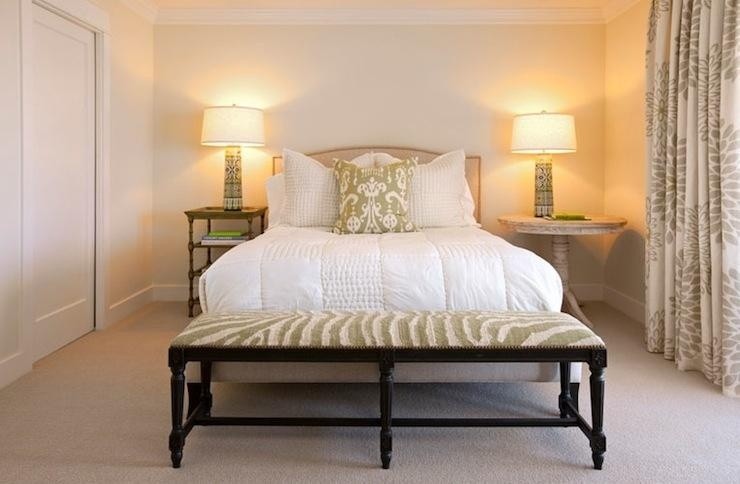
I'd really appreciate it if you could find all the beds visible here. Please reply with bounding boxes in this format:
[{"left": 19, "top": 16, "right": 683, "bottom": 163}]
[{"left": 183, "top": 144, "right": 583, "bottom": 418}]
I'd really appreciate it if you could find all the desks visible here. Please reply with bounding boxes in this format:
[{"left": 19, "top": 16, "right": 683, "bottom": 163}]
[{"left": 498, "top": 213, "right": 628, "bottom": 329}]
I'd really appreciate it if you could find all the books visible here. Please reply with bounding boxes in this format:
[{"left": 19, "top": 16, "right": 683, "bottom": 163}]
[
  {"left": 544, "top": 213, "right": 592, "bottom": 221},
  {"left": 198, "top": 231, "right": 252, "bottom": 245}
]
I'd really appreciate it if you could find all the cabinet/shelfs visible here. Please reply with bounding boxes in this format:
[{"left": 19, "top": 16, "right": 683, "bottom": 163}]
[{"left": 182, "top": 206, "right": 269, "bottom": 317}]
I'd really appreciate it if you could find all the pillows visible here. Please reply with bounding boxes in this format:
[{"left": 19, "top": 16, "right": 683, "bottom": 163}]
[{"left": 268, "top": 147, "right": 484, "bottom": 237}]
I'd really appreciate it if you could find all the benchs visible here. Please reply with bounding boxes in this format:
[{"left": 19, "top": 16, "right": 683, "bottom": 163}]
[{"left": 166, "top": 307, "right": 608, "bottom": 472}]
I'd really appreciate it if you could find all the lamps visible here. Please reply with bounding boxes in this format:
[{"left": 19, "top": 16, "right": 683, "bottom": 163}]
[
  {"left": 511, "top": 109, "right": 577, "bottom": 217},
  {"left": 200, "top": 103, "right": 267, "bottom": 210}
]
